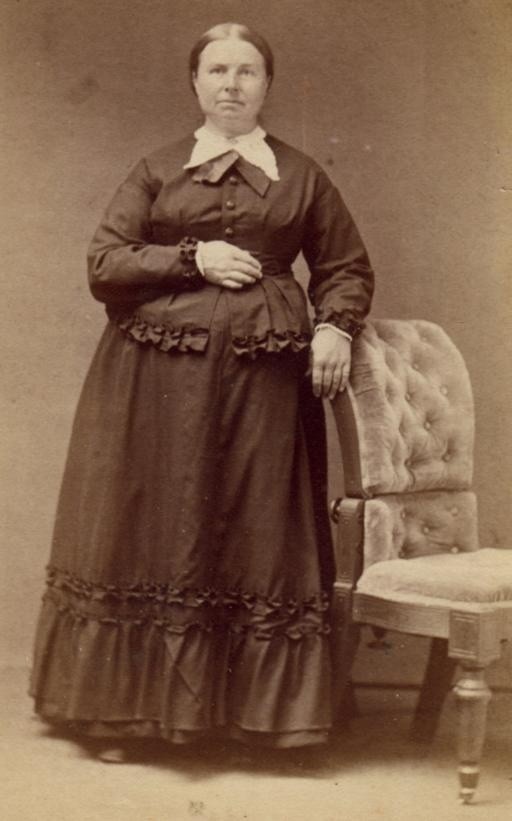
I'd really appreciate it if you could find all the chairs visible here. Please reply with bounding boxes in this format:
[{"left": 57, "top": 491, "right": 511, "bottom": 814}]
[{"left": 331, "top": 319, "right": 512, "bottom": 803}]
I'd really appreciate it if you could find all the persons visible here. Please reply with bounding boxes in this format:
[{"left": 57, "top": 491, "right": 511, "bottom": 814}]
[{"left": 22, "top": 20, "right": 376, "bottom": 769}]
